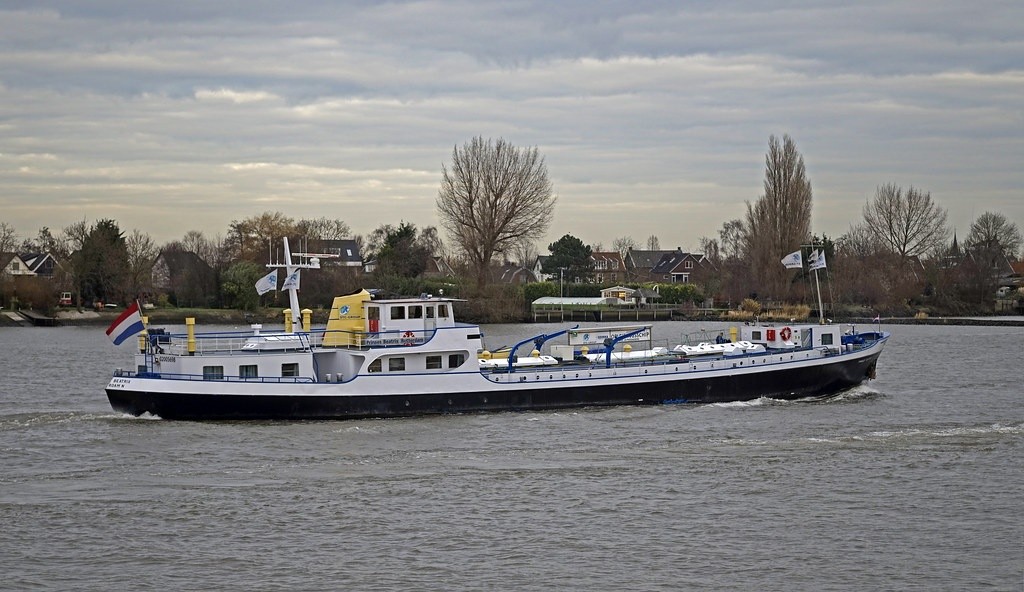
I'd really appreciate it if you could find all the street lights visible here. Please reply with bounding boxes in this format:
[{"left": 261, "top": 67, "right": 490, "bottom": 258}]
[{"left": 559, "top": 267, "right": 580, "bottom": 322}]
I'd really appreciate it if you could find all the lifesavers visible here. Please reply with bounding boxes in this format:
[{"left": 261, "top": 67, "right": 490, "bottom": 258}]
[
  {"left": 401, "top": 332, "right": 415, "bottom": 346},
  {"left": 781, "top": 327, "right": 792, "bottom": 340}
]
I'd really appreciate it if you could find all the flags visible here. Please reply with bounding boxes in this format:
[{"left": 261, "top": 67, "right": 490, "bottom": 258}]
[
  {"left": 872, "top": 316, "right": 879, "bottom": 323},
  {"left": 255, "top": 269, "right": 278, "bottom": 295},
  {"left": 808, "top": 249, "right": 825, "bottom": 271},
  {"left": 105, "top": 302, "right": 144, "bottom": 345},
  {"left": 781, "top": 249, "right": 802, "bottom": 268},
  {"left": 280, "top": 269, "right": 300, "bottom": 291}
]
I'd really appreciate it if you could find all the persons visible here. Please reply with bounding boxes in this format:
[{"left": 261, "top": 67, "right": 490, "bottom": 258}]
[
  {"left": 716, "top": 332, "right": 724, "bottom": 343},
  {"left": 95, "top": 300, "right": 104, "bottom": 311}
]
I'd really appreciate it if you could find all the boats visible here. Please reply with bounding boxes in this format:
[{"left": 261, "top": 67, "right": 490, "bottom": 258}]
[{"left": 105, "top": 231, "right": 889, "bottom": 426}]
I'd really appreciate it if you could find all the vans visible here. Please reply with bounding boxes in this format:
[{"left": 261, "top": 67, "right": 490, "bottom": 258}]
[{"left": 61, "top": 291, "right": 71, "bottom": 306}]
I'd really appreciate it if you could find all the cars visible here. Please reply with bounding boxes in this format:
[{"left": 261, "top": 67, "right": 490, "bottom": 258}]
[
  {"left": 143, "top": 301, "right": 154, "bottom": 310},
  {"left": 94, "top": 302, "right": 117, "bottom": 312}
]
